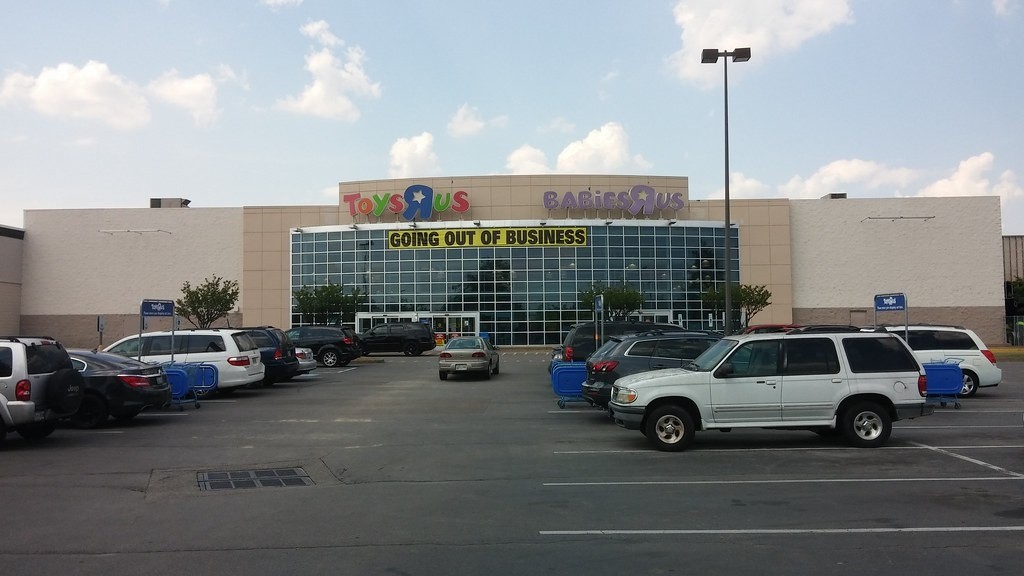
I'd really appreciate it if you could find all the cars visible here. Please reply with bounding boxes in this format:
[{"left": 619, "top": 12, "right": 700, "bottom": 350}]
[
  {"left": 548, "top": 345, "right": 563, "bottom": 373},
  {"left": 437, "top": 338, "right": 501, "bottom": 382},
  {"left": 64, "top": 344, "right": 171, "bottom": 427},
  {"left": 294, "top": 346, "right": 317, "bottom": 376}
]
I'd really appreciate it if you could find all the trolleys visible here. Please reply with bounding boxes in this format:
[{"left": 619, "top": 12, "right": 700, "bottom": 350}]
[
  {"left": 160, "top": 360, "right": 220, "bottom": 411},
  {"left": 921, "top": 357, "right": 965, "bottom": 409},
  {"left": 550, "top": 359, "right": 587, "bottom": 410}
]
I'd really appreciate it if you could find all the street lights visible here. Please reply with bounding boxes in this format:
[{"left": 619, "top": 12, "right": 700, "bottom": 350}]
[{"left": 699, "top": 48, "right": 752, "bottom": 336}]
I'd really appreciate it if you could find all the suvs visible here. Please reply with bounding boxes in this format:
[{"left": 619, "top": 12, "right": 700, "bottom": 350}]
[
  {"left": 183, "top": 326, "right": 300, "bottom": 391},
  {"left": 580, "top": 328, "right": 776, "bottom": 411},
  {"left": 861, "top": 323, "right": 1004, "bottom": 399},
  {"left": 561, "top": 321, "right": 687, "bottom": 362},
  {"left": 608, "top": 326, "right": 935, "bottom": 452},
  {"left": 734, "top": 321, "right": 802, "bottom": 336},
  {"left": 0, "top": 335, "right": 85, "bottom": 440},
  {"left": 360, "top": 321, "right": 436, "bottom": 356},
  {"left": 284, "top": 325, "right": 364, "bottom": 368},
  {"left": 101, "top": 327, "right": 265, "bottom": 400}
]
